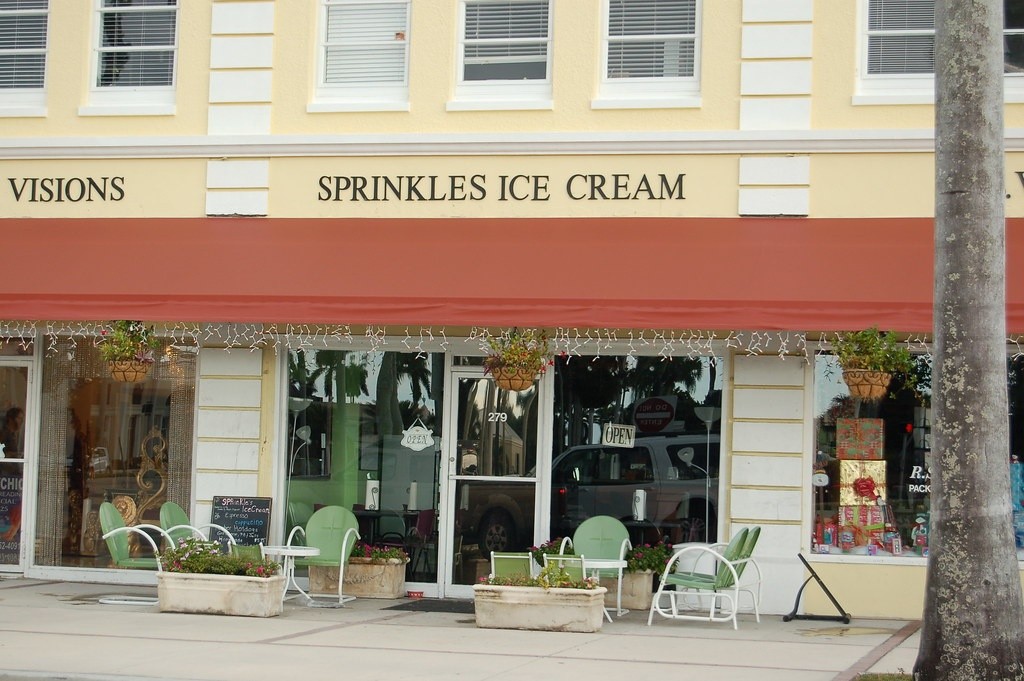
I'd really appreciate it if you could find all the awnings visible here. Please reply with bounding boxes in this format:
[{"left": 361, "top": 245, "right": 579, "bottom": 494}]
[{"left": 0, "top": 217, "right": 1024, "bottom": 336}]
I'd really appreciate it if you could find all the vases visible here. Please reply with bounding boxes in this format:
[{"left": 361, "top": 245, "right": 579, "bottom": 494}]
[
  {"left": 156, "top": 572, "right": 285, "bottom": 617},
  {"left": 472, "top": 584, "right": 608, "bottom": 634},
  {"left": 308, "top": 558, "right": 408, "bottom": 599}
]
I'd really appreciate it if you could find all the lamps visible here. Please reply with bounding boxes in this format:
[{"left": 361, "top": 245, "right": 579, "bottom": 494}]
[
  {"left": 678, "top": 407, "right": 724, "bottom": 542},
  {"left": 286, "top": 397, "right": 314, "bottom": 519}
]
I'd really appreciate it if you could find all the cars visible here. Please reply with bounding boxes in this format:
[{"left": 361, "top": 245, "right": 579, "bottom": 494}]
[
  {"left": 454, "top": 433, "right": 720, "bottom": 567},
  {"left": 63, "top": 446, "right": 112, "bottom": 485}
]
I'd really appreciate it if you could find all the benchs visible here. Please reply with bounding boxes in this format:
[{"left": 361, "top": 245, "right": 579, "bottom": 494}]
[{"left": 647, "top": 525, "right": 763, "bottom": 626}]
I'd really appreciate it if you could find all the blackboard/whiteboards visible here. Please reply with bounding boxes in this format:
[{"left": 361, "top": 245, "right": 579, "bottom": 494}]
[{"left": 209, "top": 496, "right": 272, "bottom": 553}]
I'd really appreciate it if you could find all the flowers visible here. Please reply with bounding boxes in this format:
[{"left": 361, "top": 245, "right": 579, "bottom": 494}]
[
  {"left": 154, "top": 536, "right": 281, "bottom": 577},
  {"left": 621, "top": 541, "right": 678, "bottom": 573},
  {"left": 350, "top": 543, "right": 408, "bottom": 563},
  {"left": 479, "top": 563, "right": 599, "bottom": 590},
  {"left": 529, "top": 539, "right": 573, "bottom": 567}
]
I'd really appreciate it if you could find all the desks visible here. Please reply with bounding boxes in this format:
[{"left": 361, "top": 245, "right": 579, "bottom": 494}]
[{"left": 261, "top": 546, "right": 320, "bottom": 605}]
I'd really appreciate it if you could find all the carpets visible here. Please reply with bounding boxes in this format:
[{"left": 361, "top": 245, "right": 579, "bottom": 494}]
[{"left": 380, "top": 599, "right": 475, "bottom": 614}]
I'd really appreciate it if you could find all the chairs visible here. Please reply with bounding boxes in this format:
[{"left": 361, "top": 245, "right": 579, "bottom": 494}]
[
  {"left": 287, "top": 505, "right": 362, "bottom": 605},
  {"left": 541, "top": 516, "right": 632, "bottom": 619},
  {"left": 160, "top": 501, "right": 236, "bottom": 548},
  {"left": 543, "top": 552, "right": 613, "bottom": 623},
  {"left": 490, "top": 551, "right": 531, "bottom": 581},
  {"left": 99, "top": 502, "right": 178, "bottom": 605}
]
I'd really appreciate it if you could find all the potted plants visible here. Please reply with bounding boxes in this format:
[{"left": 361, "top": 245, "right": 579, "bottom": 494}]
[
  {"left": 95, "top": 321, "right": 160, "bottom": 384},
  {"left": 481, "top": 328, "right": 554, "bottom": 389},
  {"left": 829, "top": 326, "right": 914, "bottom": 403}
]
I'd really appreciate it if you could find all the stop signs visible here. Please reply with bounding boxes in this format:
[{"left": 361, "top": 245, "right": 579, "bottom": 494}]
[{"left": 633, "top": 398, "right": 674, "bottom": 433}]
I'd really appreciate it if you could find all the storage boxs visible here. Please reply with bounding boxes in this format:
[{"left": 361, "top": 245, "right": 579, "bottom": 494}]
[{"left": 836, "top": 418, "right": 887, "bottom": 545}]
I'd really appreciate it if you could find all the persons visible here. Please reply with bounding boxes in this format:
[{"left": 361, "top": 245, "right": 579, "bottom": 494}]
[{"left": 0, "top": 408, "right": 24, "bottom": 477}]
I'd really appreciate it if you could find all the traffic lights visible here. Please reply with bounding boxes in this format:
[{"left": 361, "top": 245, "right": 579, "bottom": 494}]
[{"left": 899, "top": 422, "right": 914, "bottom": 434}]
